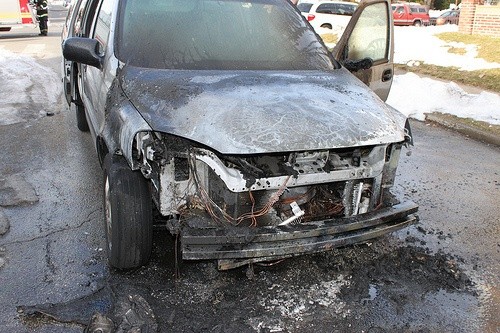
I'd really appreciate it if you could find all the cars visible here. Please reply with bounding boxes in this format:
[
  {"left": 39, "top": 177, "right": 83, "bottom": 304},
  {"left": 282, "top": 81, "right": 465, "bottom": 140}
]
[
  {"left": 61, "top": 0, "right": 423, "bottom": 272},
  {"left": 390, "top": 4, "right": 430, "bottom": 27},
  {"left": 296, "top": 0, "right": 376, "bottom": 30},
  {"left": 429, "top": 10, "right": 459, "bottom": 27}
]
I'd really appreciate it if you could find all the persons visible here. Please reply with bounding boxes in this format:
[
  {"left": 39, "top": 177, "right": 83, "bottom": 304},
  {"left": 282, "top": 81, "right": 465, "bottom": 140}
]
[
  {"left": 269, "top": 0, "right": 304, "bottom": 43},
  {"left": 29, "top": 0, "right": 48, "bottom": 36}
]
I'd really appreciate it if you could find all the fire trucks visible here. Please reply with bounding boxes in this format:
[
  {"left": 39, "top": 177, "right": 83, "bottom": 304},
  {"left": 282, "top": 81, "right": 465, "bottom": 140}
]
[{"left": 0, "top": 0, "right": 39, "bottom": 32}]
